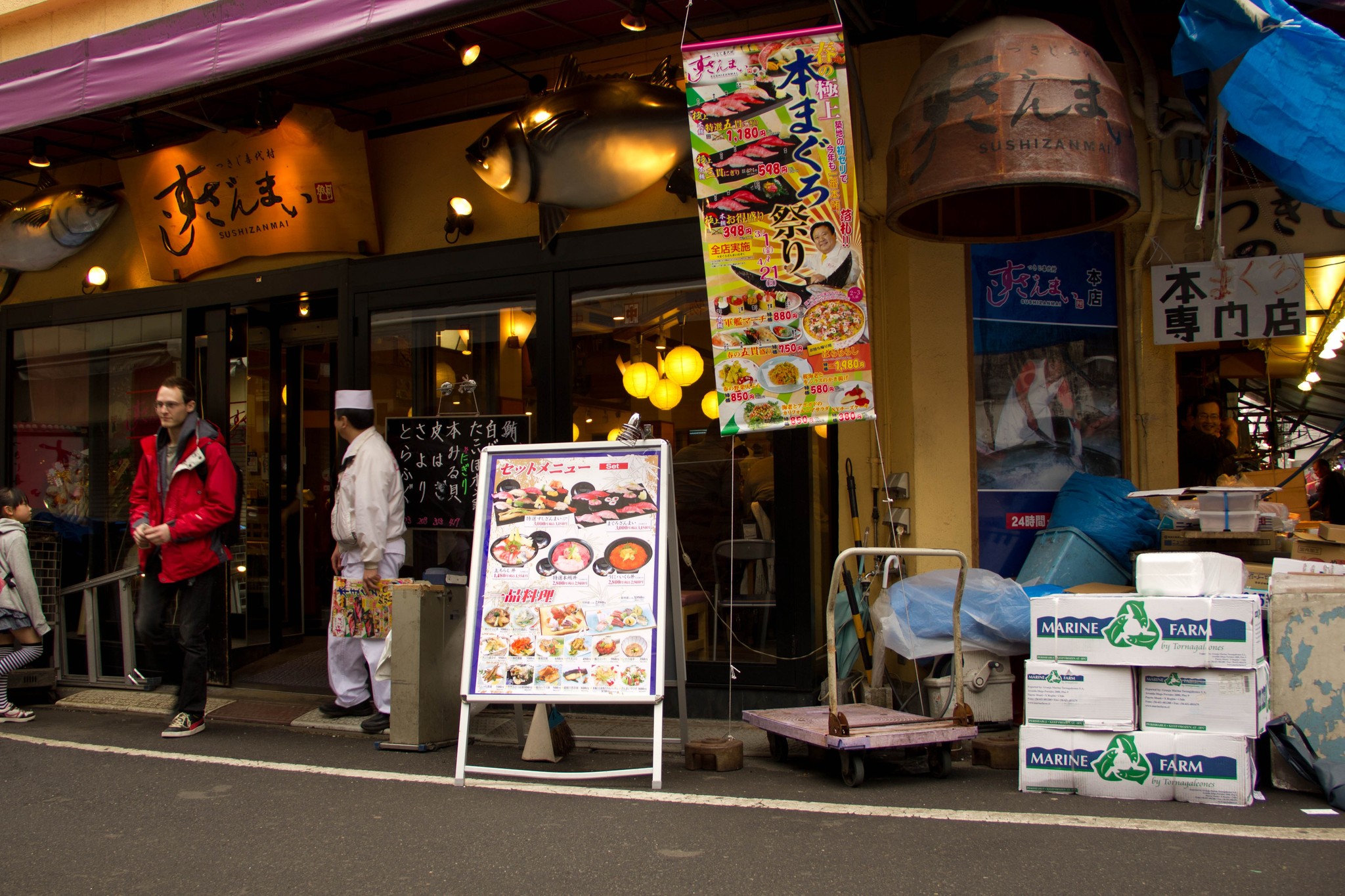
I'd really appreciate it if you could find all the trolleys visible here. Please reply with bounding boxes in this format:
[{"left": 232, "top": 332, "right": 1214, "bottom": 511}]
[{"left": 740, "top": 544, "right": 981, "bottom": 784}]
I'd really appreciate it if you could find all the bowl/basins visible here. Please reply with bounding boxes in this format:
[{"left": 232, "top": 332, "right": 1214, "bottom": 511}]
[
  {"left": 605, "top": 537, "right": 653, "bottom": 574},
  {"left": 802, "top": 299, "right": 865, "bottom": 350},
  {"left": 548, "top": 538, "right": 594, "bottom": 575},
  {"left": 490, "top": 534, "right": 538, "bottom": 568},
  {"left": 620, "top": 636, "right": 647, "bottom": 657}
]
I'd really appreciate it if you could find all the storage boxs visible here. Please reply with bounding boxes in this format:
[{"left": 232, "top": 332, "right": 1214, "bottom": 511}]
[{"left": 1011, "top": 467, "right": 1344, "bottom": 810}]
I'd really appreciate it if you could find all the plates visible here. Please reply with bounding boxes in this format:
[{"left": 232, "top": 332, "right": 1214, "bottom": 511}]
[
  {"left": 715, "top": 357, "right": 759, "bottom": 394},
  {"left": 539, "top": 601, "right": 589, "bottom": 636},
  {"left": 734, "top": 395, "right": 791, "bottom": 432},
  {"left": 509, "top": 632, "right": 535, "bottom": 657},
  {"left": 511, "top": 607, "right": 539, "bottom": 628},
  {"left": 536, "top": 641, "right": 550, "bottom": 657},
  {"left": 572, "top": 483, "right": 658, "bottom": 529},
  {"left": 709, "top": 289, "right": 802, "bottom": 320},
  {"left": 362, "top": 609, "right": 376, "bottom": 638},
  {"left": 757, "top": 355, "right": 814, "bottom": 394},
  {"left": 354, "top": 595, "right": 366, "bottom": 623},
  {"left": 482, "top": 666, "right": 502, "bottom": 684},
  {"left": 492, "top": 487, "right": 574, "bottom": 526},
  {"left": 347, "top": 610, "right": 357, "bottom": 638},
  {"left": 828, "top": 379, "right": 874, "bottom": 415},
  {"left": 584, "top": 604, "right": 657, "bottom": 637},
  {"left": 712, "top": 324, "right": 803, "bottom": 350},
  {"left": 480, "top": 638, "right": 508, "bottom": 655},
  {"left": 566, "top": 637, "right": 591, "bottom": 657}
]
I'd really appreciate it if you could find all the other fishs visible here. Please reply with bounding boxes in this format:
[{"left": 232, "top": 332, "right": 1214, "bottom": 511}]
[
  {"left": 0, "top": 170, "right": 121, "bottom": 304},
  {"left": 465, "top": 52, "right": 694, "bottom": 251}
]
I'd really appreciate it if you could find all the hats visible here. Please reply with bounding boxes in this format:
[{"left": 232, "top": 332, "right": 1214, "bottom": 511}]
[{"left": 334, "top": 389, "right": 374, "bottom": 410}]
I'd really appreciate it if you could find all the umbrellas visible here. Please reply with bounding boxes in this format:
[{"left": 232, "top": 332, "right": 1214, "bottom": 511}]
[{"left": 834, "top": 526, "right": 874, "bottom": 680}]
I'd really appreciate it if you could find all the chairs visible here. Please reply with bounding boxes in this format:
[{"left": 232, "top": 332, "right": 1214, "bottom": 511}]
[{"left": 714, "top": 538, "right": 776, "bottom": 660}]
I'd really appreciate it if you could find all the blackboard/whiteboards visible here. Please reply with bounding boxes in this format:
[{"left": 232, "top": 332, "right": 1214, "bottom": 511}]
[{"left": 384, "top": 414, "right": 531, "bottom": 532}]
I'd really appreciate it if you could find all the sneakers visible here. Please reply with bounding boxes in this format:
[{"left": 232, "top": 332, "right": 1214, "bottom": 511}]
[
  {"left": 0, "top": 703, "right": 36, "bottom": 723},
  {"left": 360, "top": 711, "right": 390, "bottom": 730},
  {"left": 320, "top": 699, "right": 373, "bottom": 716},
  {"left": 161, "top": 712, "right": 206, "bottom": 737}
]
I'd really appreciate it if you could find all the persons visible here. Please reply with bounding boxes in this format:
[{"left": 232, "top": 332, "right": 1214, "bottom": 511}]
[
  {"left": 317, "top": 389, "right": 408, "bottom": 734},
  {"left": 0, "top": 486, "right": 51, "bottom": 724},
  {"left": 1308, "top": 458, "right": 1345, "bottom": 525},
  {"left": 670, "top": 420, "right": 775, "bottom": 599},
  {"left": 1175, "top": 396, "right": 1241, "bottom": 490},
  {"left": 129, "top": 375, "right": 242, "bottom": 739},
  {"left": 786, "top": 218, "right": 862, "bottom": 290},
  {"left": 996, "top": 350, "right": 1080, "bottom": 454}
]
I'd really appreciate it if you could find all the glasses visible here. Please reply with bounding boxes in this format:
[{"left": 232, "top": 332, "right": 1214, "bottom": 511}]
[
  {"left": 1197, "top": 414, "right": 1220, "bottom": 419},
  {"left": 153, "top": 400, "right": 185, "bottom": 409}
]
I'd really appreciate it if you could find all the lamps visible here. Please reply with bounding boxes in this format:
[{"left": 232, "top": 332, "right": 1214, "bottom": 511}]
[
  {"left": 29, "top": 136, "right": 118, "bottom": 167},
  {"left": 300, "top": 302, "right": 309, "bottom": 312},
  {"left": 443, "top": 195, "right": 474, "bottom": 244},
  {"left": 612, "top": 302, "right": 719, "bottom": 420},
  {"left": 436, "top": 330, "right": 472, "bottom": 405},
  {"left": 884, "top": 13, "right": 1143, "bottom": 246},
  {"left": 620, "top": 0, "right": 706, "bottom": 45},
  {"left": 572, "top": 411, "right": 623, "bottom": 442},
  {"left": 441, "top": 30, "right": 549, "bottom": 93},
  {"left": 81, "top": 265, "right": 109, "bottom": 295},
  {"left": 524, "top": 402, "right": 532, "bottom": 417}
]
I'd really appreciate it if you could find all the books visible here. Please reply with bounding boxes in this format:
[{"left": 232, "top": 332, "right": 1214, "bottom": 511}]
[{"left": 329, "top": 575, "right": 415, "bottom": 639}]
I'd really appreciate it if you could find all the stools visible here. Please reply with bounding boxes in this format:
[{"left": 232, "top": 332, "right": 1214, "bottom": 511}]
[{"left": 681, "top": 589, "right": 709, "bottom": 662}]
